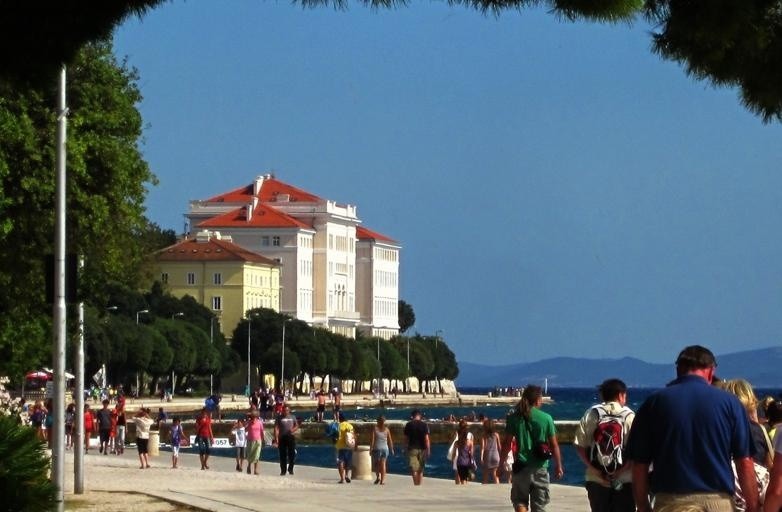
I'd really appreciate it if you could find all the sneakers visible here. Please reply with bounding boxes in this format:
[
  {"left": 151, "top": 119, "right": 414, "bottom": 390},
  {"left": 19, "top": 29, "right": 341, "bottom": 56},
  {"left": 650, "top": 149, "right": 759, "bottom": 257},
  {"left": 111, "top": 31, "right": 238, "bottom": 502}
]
[
  {"left": 140, "top": 465, "right": 150, "bottom": 469},
  {"left": 280, "top": 469, "right": 294, "bottom": 475},
  {"left": 100, "top": 447, "right": 123, "bottom": 456},
  {"left": 374, "top": 479, "right": 380, "bottom": 485},
  {"left": 345, "top": 476, "right": 350, "bottom": 483}
]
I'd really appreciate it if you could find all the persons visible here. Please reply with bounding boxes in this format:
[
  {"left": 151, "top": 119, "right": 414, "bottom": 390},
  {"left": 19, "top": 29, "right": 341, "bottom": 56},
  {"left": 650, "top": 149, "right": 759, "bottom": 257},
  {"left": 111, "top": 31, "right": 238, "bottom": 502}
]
[
  {"left": 574, "top": 344, "right": 782, "bottom": 512},
  {"left": 495, "top": 382, "right": 564, "bottom": 512},
  {"left": 333, "top": 414, "right": 354, "bottom": 484},
  {"left": 448, "top": 386, "right": 565, "bottom": 484},
  {"left": 0, "top": 384, "right": 128, "bottom": 455},
  {"left": 131, "top": 387, "right": 339, "bottom": 478},
  {"left": 368, "top": 386, "right": 444, "bottom": 399},
  {"left": 369, "top": 415, "right": 395, "bottom": 485},
  {"left": 403, "top": 410, "right": 430, "bottom": 485}
]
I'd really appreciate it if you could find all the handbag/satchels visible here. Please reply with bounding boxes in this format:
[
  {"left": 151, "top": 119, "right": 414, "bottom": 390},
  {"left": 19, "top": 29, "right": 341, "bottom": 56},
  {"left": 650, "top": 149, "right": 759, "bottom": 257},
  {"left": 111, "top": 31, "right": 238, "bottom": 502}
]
[
  {"left": 344, "top": 431, "right": 356, "bottom": 449},
  {"left": 535, "top": 439, "right": 553, "bottom": 461},
  {"left": 728, "top": 454, "right": 770, "bottom": 511},
  {"left": 236, "top": 464, "right": 259, "bottom": 475},
  {"left": 194, "top": 436, "right": 199, "bottom": 443},
  {"left": 471, "top": 458, "right": 477, "bottom": 471},
  {"left": 65, "top": 423, "right": 72, "bottom": 435}
]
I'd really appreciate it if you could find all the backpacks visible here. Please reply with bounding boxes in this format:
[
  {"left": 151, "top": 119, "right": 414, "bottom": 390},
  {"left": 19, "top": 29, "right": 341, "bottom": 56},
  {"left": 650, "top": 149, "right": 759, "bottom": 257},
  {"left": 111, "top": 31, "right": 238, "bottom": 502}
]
[
  {"left": 589, "top": 403, "right": 636, "bottom": 481},
  {"left": 170, "top": 426, "right": 180, "bottom": 446}
]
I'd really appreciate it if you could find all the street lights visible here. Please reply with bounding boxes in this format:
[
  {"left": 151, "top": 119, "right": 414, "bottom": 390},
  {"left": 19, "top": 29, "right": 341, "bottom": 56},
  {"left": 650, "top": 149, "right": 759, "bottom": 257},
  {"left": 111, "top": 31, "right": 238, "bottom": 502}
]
[
  {"left": 107, "top": 306, "right": 118, "bottom": 312},
  {"left": 435, "top": 329, "right": 443, "bottom": 394},
  {"left": 407, "top": 325, "right": 417, "bottom": 392},
  {"left": 377, "top": 326, "right": 383, "bottom": 394},
  {"left": 171, "top": 312, "right": 185, "bottom": 400},
  {"left": 282, "top": 318, "right": 293, "bottom": 390},
  {"left": 247, "top": 313, "right": 261, "bottom": 394},
  {"left": 210, "top": 314, "right": 222, "bottom": 396},
  {"left": 135, "top": 310, "right": 150, "bottom": 397}
]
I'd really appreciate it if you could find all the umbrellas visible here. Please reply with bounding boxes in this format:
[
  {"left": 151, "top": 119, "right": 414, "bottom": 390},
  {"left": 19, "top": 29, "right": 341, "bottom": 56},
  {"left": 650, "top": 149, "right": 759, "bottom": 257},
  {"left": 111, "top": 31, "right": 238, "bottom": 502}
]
[{"left": 27, "top": 367, "right": 75, "bottom": 381}]
